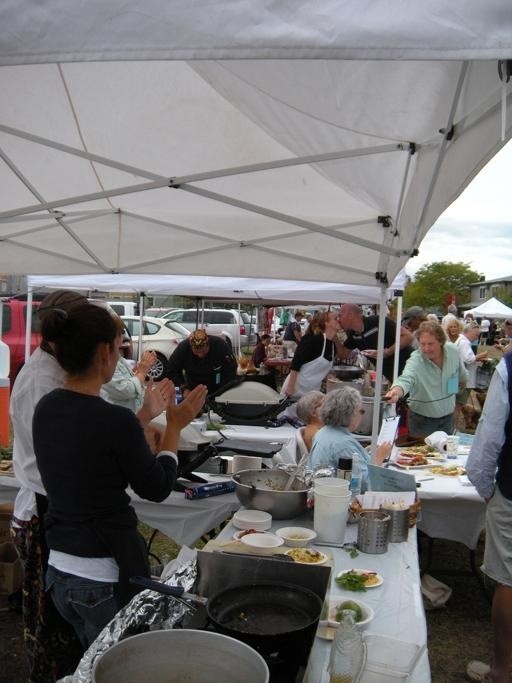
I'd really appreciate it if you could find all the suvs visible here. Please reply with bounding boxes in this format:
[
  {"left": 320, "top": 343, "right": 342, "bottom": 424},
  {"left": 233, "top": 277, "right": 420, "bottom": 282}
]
[
  {"left": 85, "top": 297, "right": 135, "bottom": 361},
  {"left": 162, "top": 308, "right": 248, "bottom": 354},
  {"left": 117, "top": 314, "right": 191, "bottom": 382}
]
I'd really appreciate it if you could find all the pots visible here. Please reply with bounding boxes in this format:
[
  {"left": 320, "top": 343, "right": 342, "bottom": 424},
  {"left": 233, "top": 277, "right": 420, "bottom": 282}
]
[
  {"left": 129, "top": 572, "right": 323, "bottom": 634},
  {"left": 214, "top": 379, "right": 283, "bottom": 418},
  {"left": 89, "top": 629, "right": 269, "bottom": 683},
  {"left": 326, "top": 364, "right": 385, "bottom": 437}
]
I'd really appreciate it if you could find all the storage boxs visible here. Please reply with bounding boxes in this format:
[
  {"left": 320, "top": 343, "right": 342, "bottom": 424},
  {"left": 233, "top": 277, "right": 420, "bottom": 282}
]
[
  {"left": 0, "top": 541, "right": 24, "bottom": 596},
  {"left": 0, "top": 503, "right": 14, "bottom": 543}
]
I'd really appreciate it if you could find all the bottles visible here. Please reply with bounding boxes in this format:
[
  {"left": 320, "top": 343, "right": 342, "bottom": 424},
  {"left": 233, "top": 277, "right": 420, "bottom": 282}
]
[
  {"left": 351, "top": 452, "right": 361, "bottom": 501},
  {"left": 175, "top": 386, "right": 182, "bottom": 404},
  {"left": 327, "top": 610, "right": 367, "bottom": 683},
  {"left": 335, "top": 456, "right": 352, "bottom": 481}
]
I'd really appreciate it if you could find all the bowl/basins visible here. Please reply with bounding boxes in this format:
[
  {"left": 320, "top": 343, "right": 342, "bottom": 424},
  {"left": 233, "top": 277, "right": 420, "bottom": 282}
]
[
  {"left": 276, "top": 526, "right": 317, "bottom": 546},
  {"left": 231, "top": 467, "right": 313, "bottom": 520}
]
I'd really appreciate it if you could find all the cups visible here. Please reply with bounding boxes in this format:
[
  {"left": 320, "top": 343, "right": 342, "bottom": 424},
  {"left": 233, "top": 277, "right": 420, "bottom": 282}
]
[
  {"left": 314, "top": 485, "right": 352, "bottom": 544},
  {"left": 190, "top": 420, "right": 206, "bottom": 435},
  {"left": 313, "top": 476, "right": 349, "bottom": 488},
  {"left": 445, "top": 435, "right": 459, "bottom": 459}
]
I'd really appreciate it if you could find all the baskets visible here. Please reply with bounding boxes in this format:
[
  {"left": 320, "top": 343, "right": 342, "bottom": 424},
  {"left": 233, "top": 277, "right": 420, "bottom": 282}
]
[
  {"left": 357, "top": 511, "right": 391, "bottom": 554},
  {"left": 380, "top": 503, "right": 409, "bottom": 543}
]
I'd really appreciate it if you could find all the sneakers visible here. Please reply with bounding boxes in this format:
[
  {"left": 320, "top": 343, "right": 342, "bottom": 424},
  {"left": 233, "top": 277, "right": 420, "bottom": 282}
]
[{"left": 467, "top": 659, "right": 496, "bottom": 682}]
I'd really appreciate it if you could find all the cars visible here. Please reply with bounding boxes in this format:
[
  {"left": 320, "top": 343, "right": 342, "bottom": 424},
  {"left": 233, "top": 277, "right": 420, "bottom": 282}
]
[
  {"left": 2, "top": 300, "right": 44, "bottom": 391},
  {"left": 144, "top": 304, "right": 183, "bottom": 324},
  {"left": 238, "top": 307, "right": 309, "bottom": 347}
]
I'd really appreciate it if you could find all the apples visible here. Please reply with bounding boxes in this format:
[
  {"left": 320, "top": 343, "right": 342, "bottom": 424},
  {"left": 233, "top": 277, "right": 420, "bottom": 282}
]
[{"left": 336, "top": 601, "right": 362, "bottom": 623}]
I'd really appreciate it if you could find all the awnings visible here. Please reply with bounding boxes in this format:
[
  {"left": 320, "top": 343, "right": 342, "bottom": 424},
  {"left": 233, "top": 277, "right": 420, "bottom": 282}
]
[{"left": 463, "top": 297, "right": 511, "bottom": 320}]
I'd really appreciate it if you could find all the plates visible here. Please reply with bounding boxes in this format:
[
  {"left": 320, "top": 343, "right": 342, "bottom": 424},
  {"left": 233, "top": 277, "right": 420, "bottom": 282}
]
[
  {"left": 338, "top": 567, "right": 383, "bottom": 588},
  {"left": 284, "top": 548, "right": 328, "bottom": 564},
  {"left": 328, "top": 592, "right": 373, "bottom": 628},
  {"left": 397, "top": 450, "right": 467, "bottom": 477},
  {"left": 233, "top": 509, "right": 272, "bottom": 530},
  {"left": 242, "top": 533, "right": 284, "bottom": 548},
  {"left": 234, "top": 530, "right": 274, "bottom": 539},
  {"left": 316, "top": 621, "right": 344, "bottom": 640},
  {"left": 478, "top": 357, "right": 493, "bottom": 360},
  {"left": 195, "top": 456, "right": 220, "bottom": 473},
  {"left": 232, "top": 453, "right": 263, "bottom": 474}
]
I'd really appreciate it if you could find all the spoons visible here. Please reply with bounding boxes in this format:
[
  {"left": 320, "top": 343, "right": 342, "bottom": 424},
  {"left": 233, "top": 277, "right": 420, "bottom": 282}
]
[{"left": 313, "top": 541, "right": 358, "bottom": 550}]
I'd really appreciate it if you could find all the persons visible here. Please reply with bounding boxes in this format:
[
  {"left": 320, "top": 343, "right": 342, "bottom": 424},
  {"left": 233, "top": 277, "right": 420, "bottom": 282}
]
[
  {"left": 98, "top": 346, "right": 158, "bottom": 414},
  {"left": 462, "top": 354, "right": 512, "bottom": 682},
  {"left": 296, "top": 391, "right": 325, "bottom": 451},
  {"left": 31, "top": 301, "right": 210, "bottom": 678},
  {"left": 252, "top": 304, "right": 392, "bottom": 417},
  {"left": 309, "top": 385, "right": 391, "bottom": 489},
  {"left": 402, "top": 306, "right": 428, "bottom": 349},
  {"left": 3, "top": 290, "right": 95, "bottom": 675},
  {"left": 162, "top": 328, "right": 236, "bottom": 412},
  {"left": 442, "top": 304, "right": 511, "bottom": 409},
  {"left": 335, "top": 304, "right": 414, "bottom": 382},
  {"left": 385, "top": 321, "right": 467, "bottom": 440}
]
